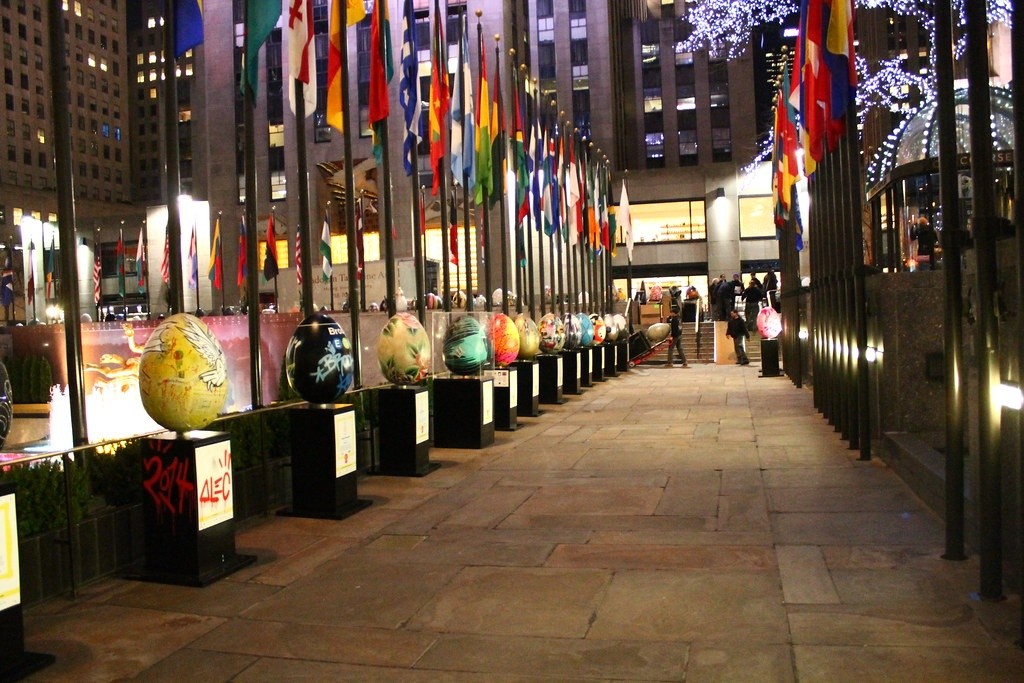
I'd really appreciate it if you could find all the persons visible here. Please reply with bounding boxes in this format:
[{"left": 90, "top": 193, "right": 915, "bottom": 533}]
[
  {"left": 664, "top": 309, "right": 688, "bottom": 367},
  {"left": 709, "top": 269, "right": 778, "bottom": 365},
  {"left": 910, "top": 217, "right": 938, "bottom": 271}
]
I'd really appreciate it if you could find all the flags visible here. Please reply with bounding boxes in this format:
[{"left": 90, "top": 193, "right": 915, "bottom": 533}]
[
  {"left": 475, "top": 25, "right": 493, "bottom": 203},
  {"left": 368, "top": 0, "right": 394, "bottom": 165},
  {"left": 449, "top": 14, "right": 476, "bottom": 192},
  {"left": 326, "top": 0, "right": 366, "bottom": 134},
  {"left": 481, "top": 203, "right": 484, "bottom": 265},
  {"left": 289, "top": 0, "right": 317, "bottom": 119},
  {"left": 618, "top": 179, "right": 634, "bottom": 263},
  {"left": 245, "top": 0, "right": 282, "bottom": 107},
  {"left": 509, "top": 62, "right": 617, "bottom": 259},
  {"left": 772, "top": 1, "right": 858, "bottom": 251},
  {"left": 419, "top": 191, "right": 426, "bottom": 236},
  {"left": 175, "top": 0, "right": 204, "bottom": 60},
  {"left": 1, "top": 173, "right": 397, "bottom": 321},
  {"left": 399, "top": 0, "right": 422, "bottom": 177},
  {"left": 489, "top": 48, "right": 508, "bottom": 209},
  {"left": 450, "top": 187, "right": 457, "bottom": 266},
  {"left": 428, "top": 7, "right": 451, "bottom": 196}
]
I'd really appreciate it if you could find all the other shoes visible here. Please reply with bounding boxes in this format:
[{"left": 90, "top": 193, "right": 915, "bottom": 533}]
[
  {"left": 681, "top": 363, "right": 687, "bottom": 368},
  {"left": 736, "top": 362, "right": 749, "bottom": 365},
  {"left": 665, "top": 364, "right": 673, "bottom": 368}
]
